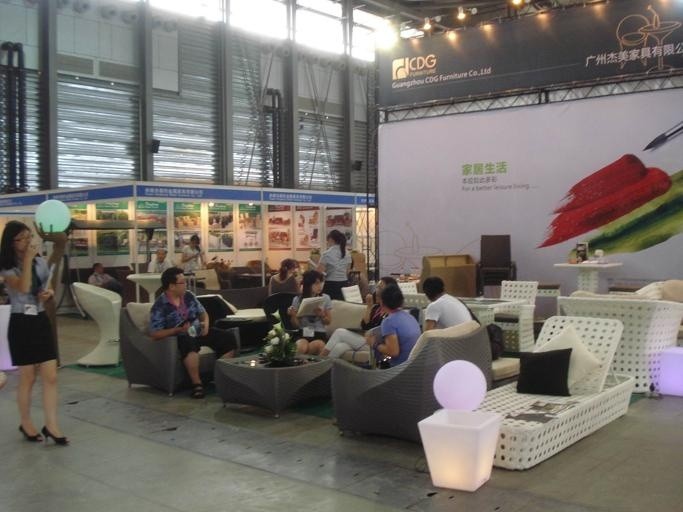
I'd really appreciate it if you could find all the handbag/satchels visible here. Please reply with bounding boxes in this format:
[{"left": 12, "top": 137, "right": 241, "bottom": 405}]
[{"left": 486, "top": 324, "right": 505, "bottom": 360}]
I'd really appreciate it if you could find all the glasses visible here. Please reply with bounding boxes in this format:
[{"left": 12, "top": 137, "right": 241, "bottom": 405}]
[{"left": 15, "top": 235, "right": 34, "bottom": 243}]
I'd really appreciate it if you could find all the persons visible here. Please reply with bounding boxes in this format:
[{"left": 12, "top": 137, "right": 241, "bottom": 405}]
[
  {"left": 148, "top": 268, "right": 235, "bottom": 399},
  {"left": 0, "top": 220, "right": 68, "bottom": 447},
  {"left": 89, "top": 262, "right": 122, "bottom": 296},
  {"left": 180, "top": 234, "right": 205, "bottom": 272},
  {"left": 267, "top": 229, "right": 475, "bottom": 368},
  {"left": 147, "top": 246, "right": 174, "bottom": 274}
]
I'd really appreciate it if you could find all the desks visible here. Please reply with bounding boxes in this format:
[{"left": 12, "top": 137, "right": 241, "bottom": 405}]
[{"left": 554, "top": 260, "right": 623, "bottom": 293}]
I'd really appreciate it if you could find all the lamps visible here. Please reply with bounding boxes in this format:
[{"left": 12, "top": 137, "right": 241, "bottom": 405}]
[
  {"left": 417, "top": 360, "right": 500, "bottom": 494},
  {"left": 424, "top": 0, "right": 522, "bottom": 40}
]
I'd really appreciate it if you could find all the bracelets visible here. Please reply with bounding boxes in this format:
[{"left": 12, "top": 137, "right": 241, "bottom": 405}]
[{"left": 173, "top": 327, "right": 179, "bottom": 334}]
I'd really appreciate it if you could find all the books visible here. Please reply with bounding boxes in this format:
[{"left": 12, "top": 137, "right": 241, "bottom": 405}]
[
  {"left": 575, "top": 241, "right": 588, "bottom": 264},
  {"left": 44, "top": 263, "right": 56, "bottom": 289}
]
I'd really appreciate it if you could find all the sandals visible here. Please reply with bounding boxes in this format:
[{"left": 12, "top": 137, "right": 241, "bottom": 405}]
[{"left": 193, "top": 383, "right": 204, "bottom": 399}]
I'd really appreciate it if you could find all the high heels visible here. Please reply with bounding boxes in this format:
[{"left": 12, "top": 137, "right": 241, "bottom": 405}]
[
  {"left": 19, "top": 424, "right": 43, "bottom": 442},
  {"left": 41, "top": 426, "right": 70, "bottom": 445}
]
